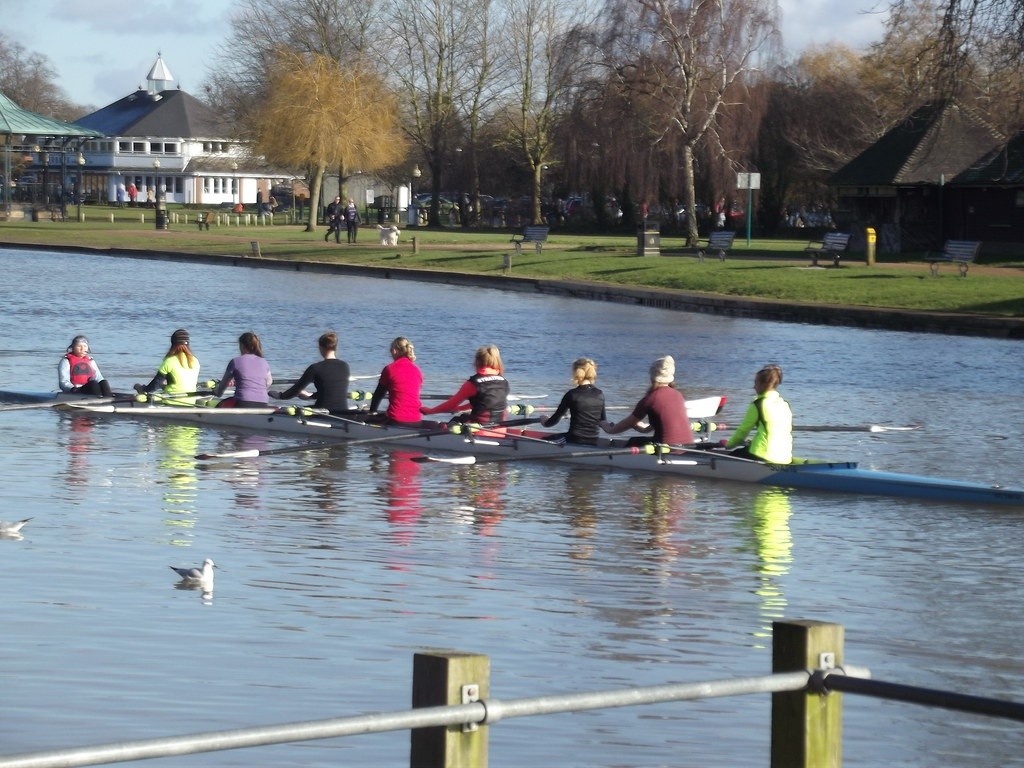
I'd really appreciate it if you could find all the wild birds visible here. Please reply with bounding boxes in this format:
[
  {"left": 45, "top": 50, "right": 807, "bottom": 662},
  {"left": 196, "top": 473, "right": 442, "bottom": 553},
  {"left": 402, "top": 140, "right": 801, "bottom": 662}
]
[
  {"left": 168, "top": 559, "right": 218, "bottom": 583},
  {"left": 0, "top": 518, "right": 33, "bottom": 534}
]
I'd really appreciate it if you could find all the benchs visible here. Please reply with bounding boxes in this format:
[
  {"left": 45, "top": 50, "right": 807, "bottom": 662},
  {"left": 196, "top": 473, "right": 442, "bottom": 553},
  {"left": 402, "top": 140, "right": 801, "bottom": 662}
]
[
  {"left": 925, "top": 240, "right": 984, "bottom": 277},
  {"left": 804, "top": 232, "right": 852, "bottom": 267},
  {"left": 510, "top": 224, "right": 551, "bottom": 253},
  {"left": 694, "top": 231, "right": 737, "bottom": 257},
  {"left": 35, "top": 206, "right": 69, "bottom": 222},
  {"left": 193, "top": 212, "right": 215, "bottom": 231}
]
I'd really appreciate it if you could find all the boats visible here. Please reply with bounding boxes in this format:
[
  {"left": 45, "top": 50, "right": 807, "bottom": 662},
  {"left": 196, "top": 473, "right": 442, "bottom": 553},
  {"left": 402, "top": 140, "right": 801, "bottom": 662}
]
[{"left": 0, "top": 385, "right": 1024, "bottom": 516}]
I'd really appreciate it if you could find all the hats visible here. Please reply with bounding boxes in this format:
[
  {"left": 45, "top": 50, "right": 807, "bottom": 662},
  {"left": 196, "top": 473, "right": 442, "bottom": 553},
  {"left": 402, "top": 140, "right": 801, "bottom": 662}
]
[
  {"left": 171, "top": 330, "right": 189, "bottom": 345},
  {"left": 650, "top": 355, "right": 675, "bottom": 383}
]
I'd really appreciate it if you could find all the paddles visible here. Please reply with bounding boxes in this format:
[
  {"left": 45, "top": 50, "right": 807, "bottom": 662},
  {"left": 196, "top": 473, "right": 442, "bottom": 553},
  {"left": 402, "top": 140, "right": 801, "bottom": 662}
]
[
  {"left": 195, "top": 372, "right": 381, "bottom": 390},
  {"left": 593, "top": 421, "right": 922, "bottom": 435},
  {"left": 194, "top": 414, "right": 571, "bottom": 461},
  {"left": 458, "top": 395, "right": 729, "bottom": 420},
  {"left": 412, "top": 441, "right": 749, "bottom": 467},
  {"left": 0, "top": 390, "right": 236, "bottom": 411},
  {"left": 51, "top": 402, "right": 369, "bottom": 414},
  {"left": 348, "top": 391, "right": 550, "bottom": 402}
]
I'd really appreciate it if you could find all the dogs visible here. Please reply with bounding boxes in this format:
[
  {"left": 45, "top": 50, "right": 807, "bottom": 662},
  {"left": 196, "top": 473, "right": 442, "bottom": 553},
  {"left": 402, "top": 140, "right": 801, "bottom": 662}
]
[{"left": 377, "top": 225, "right": 401, "bottom": 246}]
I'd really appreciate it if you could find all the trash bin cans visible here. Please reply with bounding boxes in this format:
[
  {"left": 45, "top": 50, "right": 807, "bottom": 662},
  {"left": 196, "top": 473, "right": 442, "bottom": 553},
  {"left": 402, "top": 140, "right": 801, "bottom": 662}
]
[
  {"left": 408, "top": 205, "right": 417, "bottom": 225},
  {"left": 638, "top": 231, "right": 661, "bottom": 257},
  {"left": 24, "top": 208, "right": 38, "bottom": 222},
  {"left": 156, "top": 210, "right": 167, "bottom": 229}
]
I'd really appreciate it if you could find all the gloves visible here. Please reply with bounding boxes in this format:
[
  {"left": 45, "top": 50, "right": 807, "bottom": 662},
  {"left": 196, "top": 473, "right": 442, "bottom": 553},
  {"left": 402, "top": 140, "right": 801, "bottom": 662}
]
[
  {"left": 134, "top": 384, "right": 141, "bottom": 390},
  {"left": 419, "top": 407, "right": 429, "bottom": 414},
  {"left": 268, "top": 390, "right": 281, "bottom": 399},
  {"left": 71, "top": 387, "right": 77, "bottom": 393},
  {"left": 212, "top": 378, "right": 221, "bottom": 387},
  {"left": 541, "top": 414, "right": 548, "bottom": 425},
  {"left": 720, "top": 440, "right": 727, "bottom": 445}
]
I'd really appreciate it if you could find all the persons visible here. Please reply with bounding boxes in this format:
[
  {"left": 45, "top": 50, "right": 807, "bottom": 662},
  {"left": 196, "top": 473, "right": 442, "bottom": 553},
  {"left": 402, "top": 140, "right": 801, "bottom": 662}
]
[
  {"left": 343, "top": 198, "right": 362, "bottom": 243},
  {"left": 642, "top": 201, "right": 649, "bottom": 218},
  {"left": 212, "top": 333, "right": 272, "bottom": 408},
  {"left": 134, "top": 328, "right": 201, "bottom": 408},
  {"left": 540, "top": 357, "right": 606, "bottom": 447},
  {"left": 325, "top": 196, "right": 345, "bottom": 243},
  {"left": 267, "top": 332, "right": 350, "bottom": 412},
  {"left": 257, "top": 188, "right": 263, "bottom": 217},
  {"left": 127, "top": 183, "right": 137, "bottom": 202},
  {"left": 598, "top": 354, "right": 695, "bottom": 455},
  {"left": 269, "top": 196, "right": 279, "bottom": 215},
  {"left": 366, "top": 337, "right": 424, "bottom": 428},
  {"left": 58, "top": 336, "right": 113, "bottom": 397},
  {"left": 419, "top": 345, "right": 510, "bottom": 430},
  {"left": 117, "top": 188, "right": 124, "bottom": 207},
  {"left": 719, "top": 363, "right": 793, "bottom": 465},
  {"left": 146, "top": 185, "right": 154, "bottom": 208},
  {"left": 715, "top": 197, "right": 726, "bottom": 228}
]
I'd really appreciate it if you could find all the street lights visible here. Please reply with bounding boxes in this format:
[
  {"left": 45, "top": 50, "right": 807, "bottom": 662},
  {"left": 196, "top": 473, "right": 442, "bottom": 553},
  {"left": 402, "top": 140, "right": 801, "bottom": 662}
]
[
  {"left": 78, "top": 152, "right": 86, "bottom": 221},
  {"left": 41, "top": 150, "right": 51, "bottom": 205},
  {"left": 411, "top": 163, "right": 423, "bottom": 232},
  {"left": 152, "top": 158, "right": 160, "bottom": 203},
  {"left": 231, "top": 160, "right": 238, "bottom": 210}
]
